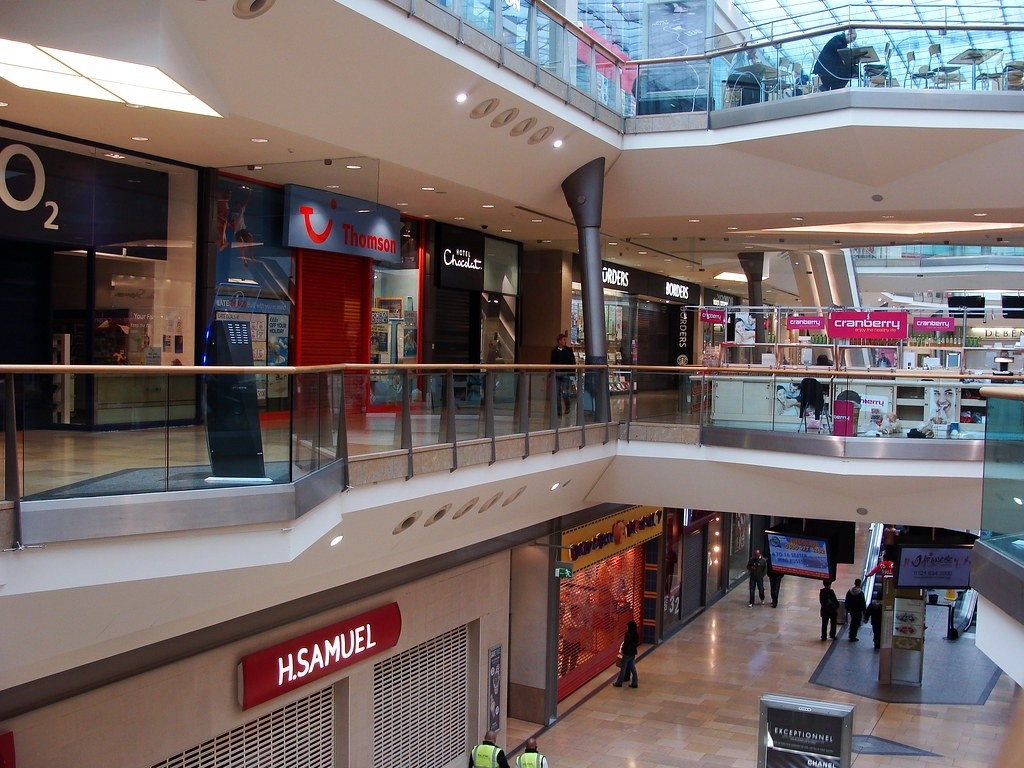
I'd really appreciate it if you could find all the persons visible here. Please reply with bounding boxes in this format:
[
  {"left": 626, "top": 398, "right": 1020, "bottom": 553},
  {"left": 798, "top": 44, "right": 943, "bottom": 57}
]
[
  {"left": 404, "top": 331, "right": 416, "bottom": 355},
  {"left": 816, "top": 354, "right": 832, "bottom": 366},
  {"left": 819, "top": 579, "right": 840, "bottom": 642},
  {"left": 747, "top": 550, "right": 767, "bottom": 607},
  {"left": 877, "top": 352, "right": 891, "bottom": 367},
  {"left": 735, "top": 314, "right": 755, "bottom": 343},
  {"left": 515, "top": 738, "right": 549, "bottom": 768},
  {"left": 864, "top": 592, "right": 883, "bottom": 650},
  {"left": 933, "top": 387, "right": 955, "bottom": 422},
  {"left": 468, "top": 730, "right": 510, "bottom": 768},
  {"left": 559, "top": 605, "right": 584, "bottom": 676},
  {"left": 549, "top": 333, "right": 576, "bottom": 418},
  {"left": 777, "top": 384, "right": 800, "bottom": 416},
  {"left": 487, "top": 339, "right": 502, "bottom": 363},
  {"left": 767, "top": 557, "right": 785, "bottom": 608},
  {"left": 865, "top": 554, "right": 895, "bottom": 588},
  {"left": 612, "top": 620, "right": 639, "bottom": 688},
  {"left": 883, "top": 524, "right": 911, "bottom": 560},
  {"left": 726, "top": 40, "right": 769, "bottom": 106},
  {"left": 845, "top": 578, "right": 866, "bottom": 642},
  {"left": 812, "top": 29, "right": 857, "bottom": 92},
  {"left": 378, "top": 332, "right": 387, "bottom": 351}
]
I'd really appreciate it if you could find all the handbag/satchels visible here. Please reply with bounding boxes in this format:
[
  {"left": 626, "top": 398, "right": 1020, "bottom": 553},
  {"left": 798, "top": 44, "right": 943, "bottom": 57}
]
[
  {"left": 616, "top": 641, "right": 624, "bottom": 667},
  {"left": 559, "top": 376, "right": 578, "bottom": 399},
  {"left": 822, "top": 593, "right": 839, "bottom": 608},
  {"left": 807, "top": 418, "right": 823, "bottom": 429}
]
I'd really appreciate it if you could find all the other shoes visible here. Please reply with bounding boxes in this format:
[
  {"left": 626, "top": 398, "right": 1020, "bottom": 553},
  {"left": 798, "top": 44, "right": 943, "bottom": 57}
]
[
  {"left": 628, "top": 684, "right": 638, "bottom": 688},
  {"left": 850, "top": 638, "right": 859, "bottom": 642},
  {"left": 773, "top": 605, "right": 776, "bottom": 608},
  {"left": 748, "top": 604, "right": 753, "bottom": 607},
  {"left": 565, "top": 408, "right": 570, "bottom": 414},
  {"left": 771, "top": 603, "right": 774, "bottom": 604},
  {"left": 822, "top": 637, "right": 827, "bottom": 640},
  {"left": 830, "top": 635, "right": 836, "bottom": 639},
  {"left": 558, "top": 409, "right": 562, "bottom": 416},
  {"left": 613, "top": 682, "right": 622, "bottom": 687},
  {"left": 761, "top": 600, "right": 765, "bottom": 605}
]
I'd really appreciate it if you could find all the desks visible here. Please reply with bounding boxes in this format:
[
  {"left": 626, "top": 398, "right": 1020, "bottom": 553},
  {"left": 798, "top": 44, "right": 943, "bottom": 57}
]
[
  {"left": 734, "top": 65, "right": 790, "bottom": 102},
  {"left": 947, "top": 49, "right": 1003, "bottom": 91},
  {"left": 837, "top": 46, "right": 880, "bottom": 87}
]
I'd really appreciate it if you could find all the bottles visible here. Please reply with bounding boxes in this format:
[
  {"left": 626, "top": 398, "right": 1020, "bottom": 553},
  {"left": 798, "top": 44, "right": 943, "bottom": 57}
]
[
  {"left": 903, "top": 334, "right": 982, "bottom": 347},
  {"left": 850, "top": 338, "right": 896, "bottom": 346},
  {"left": 767, "top": 331, "right": 776, "bottom": 343},
  {"left": 810, "top": 332, "right": 826, "bottom": 344}
]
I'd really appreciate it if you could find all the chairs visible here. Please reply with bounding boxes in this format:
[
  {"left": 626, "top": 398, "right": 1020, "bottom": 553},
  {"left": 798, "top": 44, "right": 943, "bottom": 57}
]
[
  {"left": 722, "top": 42, "right": 1024, "bottom": 108},
  {"left": 797, "top": 378, "right": 831, "bottom": 434}
]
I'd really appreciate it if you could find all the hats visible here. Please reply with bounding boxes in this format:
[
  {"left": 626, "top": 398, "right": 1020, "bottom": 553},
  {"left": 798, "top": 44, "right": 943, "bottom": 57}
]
[{"left": 823, "top": 580, "right": 831, "bottom": 586}]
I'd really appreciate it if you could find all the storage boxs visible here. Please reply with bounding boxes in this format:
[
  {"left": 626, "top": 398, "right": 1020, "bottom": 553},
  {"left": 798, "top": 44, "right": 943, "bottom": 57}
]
[{"left": 932, "top": 422, "right": 960, "bottom": 439}]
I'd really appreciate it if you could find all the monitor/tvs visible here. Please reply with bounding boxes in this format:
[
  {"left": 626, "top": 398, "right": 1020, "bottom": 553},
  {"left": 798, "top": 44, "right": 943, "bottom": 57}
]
[
  {"left": 764, "top": 518, "right": 856, "bottom": 582},
  {"left": 947, "top": 295, "right": 985, "bottom": 319},
  {"left": 1002, "top": 296, "right": 1024, "bottom": 319},
  {"left": 893, "top": 527, "right": 980, "bottom": 590}
]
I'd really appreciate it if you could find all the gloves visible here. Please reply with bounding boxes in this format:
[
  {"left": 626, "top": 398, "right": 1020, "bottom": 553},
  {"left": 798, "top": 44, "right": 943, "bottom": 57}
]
[{"left": 863, "top": 619, "right": 867, "bottom": 623}]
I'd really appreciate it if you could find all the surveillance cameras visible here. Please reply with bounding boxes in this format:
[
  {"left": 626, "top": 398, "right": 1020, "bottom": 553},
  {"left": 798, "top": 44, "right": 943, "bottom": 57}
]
[{"left": 481, "top": 225, "right": 488, "bottom": 229}]
